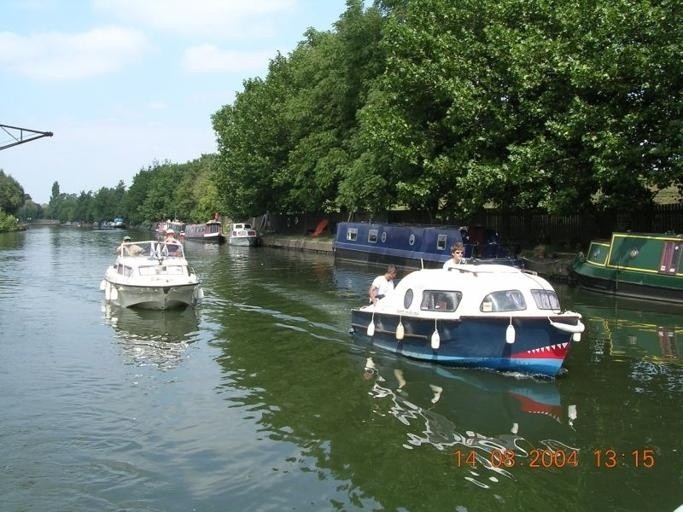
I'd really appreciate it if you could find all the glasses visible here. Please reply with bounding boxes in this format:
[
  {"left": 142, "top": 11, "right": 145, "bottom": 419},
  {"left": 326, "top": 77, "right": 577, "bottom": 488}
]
[{"left": 456, "top": 252, "right": 463, "bottom": 254}]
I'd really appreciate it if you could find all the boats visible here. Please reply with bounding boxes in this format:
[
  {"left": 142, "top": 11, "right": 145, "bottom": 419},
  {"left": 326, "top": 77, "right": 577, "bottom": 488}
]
[
  {"left": 149, "top": 216, "right": 258, "bottom": 247},
  {"left": 328, "top": 219, "right": 519, "bottom": 270},
  {"left": 569, "top": 228, "right": 683, "bottom": 307},
  {"left": 347, "top": 260, "right": 586, "bottom": 380},
  {"left": 97, "top": 240, "right": 206, "bottom": 311}
]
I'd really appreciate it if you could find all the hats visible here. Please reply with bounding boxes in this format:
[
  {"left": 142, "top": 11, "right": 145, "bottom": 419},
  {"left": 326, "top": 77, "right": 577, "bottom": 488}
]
[
  {"left": 166, "top": 229, "right": 174, "bottom": 233},
  {"left": 123, "top": 236, "right": 131, "bottom": 240}
]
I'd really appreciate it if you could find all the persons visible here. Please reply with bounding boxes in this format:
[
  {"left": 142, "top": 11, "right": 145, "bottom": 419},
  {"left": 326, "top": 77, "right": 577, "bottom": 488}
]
[
  {"left": 368, "top": 263, "right": 400, "bottom": 303},
  {"left": 117, "top": 229, "right": 181, "bottom": 256},
  {"left": 443, "top": 242, "right": 467, "bottom": 268}
]
